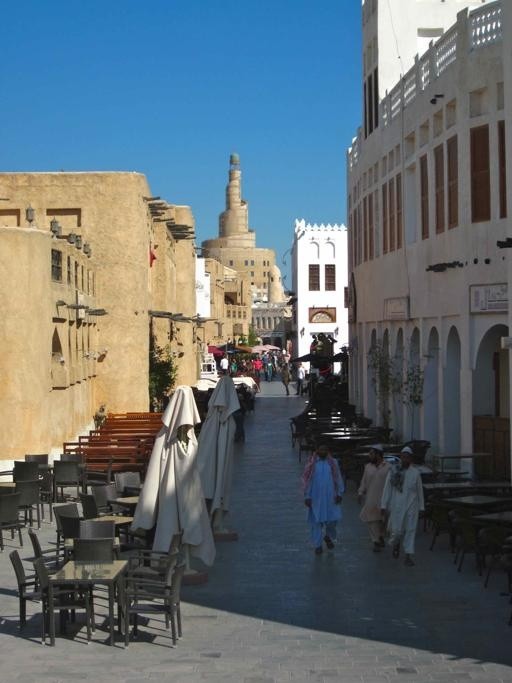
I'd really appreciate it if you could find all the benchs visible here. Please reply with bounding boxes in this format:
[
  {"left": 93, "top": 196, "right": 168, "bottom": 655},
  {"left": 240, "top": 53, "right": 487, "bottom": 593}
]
[{"left": 63, "top": 409, "right": 167, "bottom": 472}]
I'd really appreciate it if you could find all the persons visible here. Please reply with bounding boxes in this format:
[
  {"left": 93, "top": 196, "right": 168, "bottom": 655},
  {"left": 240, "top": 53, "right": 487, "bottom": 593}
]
[
  {"left": 378, "top": 444, "right": 425, "bottom": 567},
  {"left": 295, "top": 361, "right": 305, "bottom": 395},
  {"left": 357, "top": 443, "right": 393, "bottom": 551},
  {"left": 301, "top": 436, "right": 346, "bottom": 555},
  {"left": 219, "top": 350, "right": 288, "bottom": 381},
  {"left": 280, "top": 362, "right": 291, "bottom": 396}
]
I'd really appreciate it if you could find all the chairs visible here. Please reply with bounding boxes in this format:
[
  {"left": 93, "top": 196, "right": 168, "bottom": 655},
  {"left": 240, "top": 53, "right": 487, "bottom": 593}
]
[{"left": 1, "top": 454, "right": 201, "bottom": 644}]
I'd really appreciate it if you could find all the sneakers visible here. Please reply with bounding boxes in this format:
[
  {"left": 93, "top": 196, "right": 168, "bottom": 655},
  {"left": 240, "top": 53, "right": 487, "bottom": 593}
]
[
  {"left": 375, "top": 535, "right": 385, "bottom": 548},
  {"left": 403, "top": 553, "right": 415, "bottom": 567},
  {"left": 392, "top": 539, "right": 400, "bottom": 560},
  {"left": 315, "top": 545, "right": 322, "bottom": 554},
  {"left": 324, "top": 536, "right": 334, "bottom": 549},
  {"left": 373, "top": 544, "right": 382, "bottom": 553}
]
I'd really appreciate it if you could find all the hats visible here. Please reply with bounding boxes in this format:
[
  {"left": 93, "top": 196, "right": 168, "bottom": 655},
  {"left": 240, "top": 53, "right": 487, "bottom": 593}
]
[
  {"left": 401, "top": 446, "right": 413, "bottom": 455},
  {"left": 371, "top": 444, "right": 384, "bottom": 455}
]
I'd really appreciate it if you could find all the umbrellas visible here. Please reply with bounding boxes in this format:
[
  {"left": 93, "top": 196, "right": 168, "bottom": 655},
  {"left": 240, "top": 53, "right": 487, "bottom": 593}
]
[
  {"left": 193, "top": 372, "right": 240, "bottom": 528},
  {"left": 219, "top": 342, "right": 281, "bottom": 355},
  {"left": 131, "top": 383, "right": 218, "bottom": 570}
]
